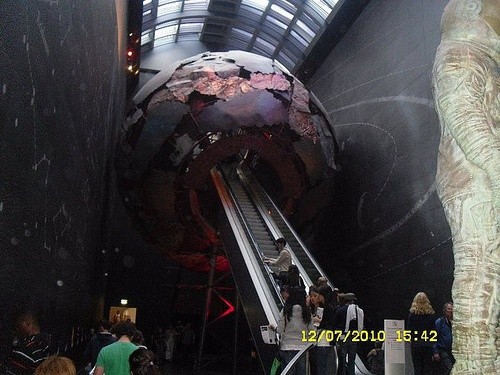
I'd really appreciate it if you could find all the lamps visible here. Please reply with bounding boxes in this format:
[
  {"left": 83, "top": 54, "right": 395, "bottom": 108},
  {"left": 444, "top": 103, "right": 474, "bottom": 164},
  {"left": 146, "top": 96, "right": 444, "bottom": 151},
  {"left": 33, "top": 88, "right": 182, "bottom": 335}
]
[{"left": 128, "top": 33, "right": 140, "bottom": 44}]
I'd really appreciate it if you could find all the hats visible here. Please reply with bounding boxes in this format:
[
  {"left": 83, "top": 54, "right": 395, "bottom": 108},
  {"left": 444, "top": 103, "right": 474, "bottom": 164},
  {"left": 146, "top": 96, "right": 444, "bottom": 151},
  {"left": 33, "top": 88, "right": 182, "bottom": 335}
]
[
  {"left": 345, "top": 293, "right": 358, "bottom": 301},
  {"left": 318, "top": 277, "right": 328, "bottom": 286}
]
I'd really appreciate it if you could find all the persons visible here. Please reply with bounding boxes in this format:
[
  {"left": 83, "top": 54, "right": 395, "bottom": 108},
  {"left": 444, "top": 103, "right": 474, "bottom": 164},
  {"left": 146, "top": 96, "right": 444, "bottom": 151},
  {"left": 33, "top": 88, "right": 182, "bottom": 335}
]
[
  {"left": 30, "top": 304, "right": 198, "bottom": 375},
  {"left": 403, "top": 291, "right": 437, "bottom": 374},
  {"left": 262, "top": 237, "right": 292, "bottom": 293},
  {"left": 366, "top": 339, "right": 385, "bottom": 375},
  {"left": 272, "top": 264, "right": 364, "bottom": 375},
  {"left": 0, "top": 306, "right": 54, "bottom": 374},
  {"left": 432, "top": 303, "right": 455, "bottom": 375},
  {"left": 428, "top": 0, "right": 500, "bottom": 375}
]
